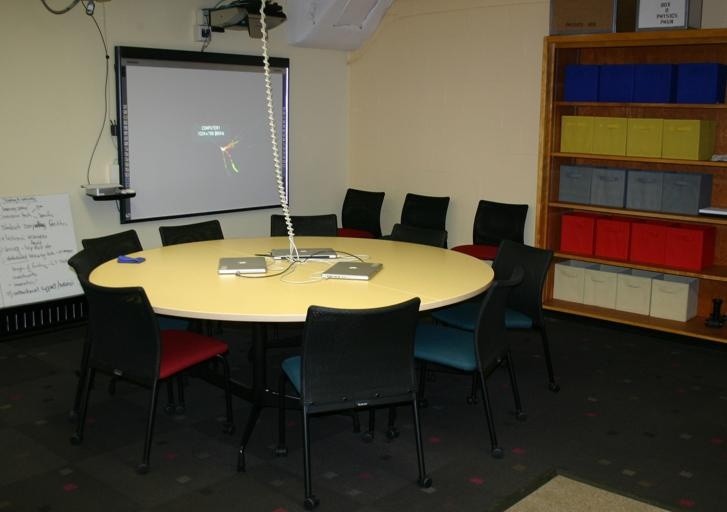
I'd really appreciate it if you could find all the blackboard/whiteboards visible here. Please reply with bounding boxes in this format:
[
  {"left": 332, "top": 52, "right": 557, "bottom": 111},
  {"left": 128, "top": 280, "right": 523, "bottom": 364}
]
[{"left": 0, "top": 191, "right": 85, "bottom": 309}]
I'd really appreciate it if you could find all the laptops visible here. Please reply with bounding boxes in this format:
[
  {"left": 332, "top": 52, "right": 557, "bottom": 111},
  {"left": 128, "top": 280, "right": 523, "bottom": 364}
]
[
  {"left": 216, "top": 255, "right": 269, "bottom": 279},
  {"left": 271, "top": 246, "right": 337, "bottom": 262},
  {"left": 322, "top": 257, "right": 385, "bottom": 283}
]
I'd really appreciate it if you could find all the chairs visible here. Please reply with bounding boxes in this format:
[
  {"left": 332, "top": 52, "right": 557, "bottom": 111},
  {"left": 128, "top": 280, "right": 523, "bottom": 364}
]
[
  {"left": 277, "top": 297, "right": 434, "bottom": 512},
  {"left": 159, "top": 219, "right": 225, "bottom": 246},
  {"left": 451, "top": 200, "right": 529, "bottom": 261},
  {"left": 82, "top": 229, "right": 144, "bottom": 263},
  {"left": 83, "top": 279, "right": 234, "bottom": 466},
  {"left": 337, "top": 188, "right": 385, "bottom": 240},
  {"left": 270, "top": 213, "right": 338, "bottom": 237},
  {"left": 387, "top": 264, "right": 527, "bottom": 460},
  {"left": 68, "top": 246, "right": 102, "bottom": 295},
  {"left": 432, "top": 239, "right": 560, "bottom": 405},
  {"left": 391, "top": 193, "right": 450, "bottom": 249}
]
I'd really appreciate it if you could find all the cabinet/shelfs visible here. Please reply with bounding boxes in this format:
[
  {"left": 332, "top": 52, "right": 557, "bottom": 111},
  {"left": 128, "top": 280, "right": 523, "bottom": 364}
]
[{"left": 534, "top": 28, "right": 727, "bottom": 344}]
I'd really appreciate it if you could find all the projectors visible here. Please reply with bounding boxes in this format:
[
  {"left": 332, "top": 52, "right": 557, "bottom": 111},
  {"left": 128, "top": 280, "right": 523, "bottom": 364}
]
[{"left": 210, "top": 1, "right": 289, "bottom": 40}]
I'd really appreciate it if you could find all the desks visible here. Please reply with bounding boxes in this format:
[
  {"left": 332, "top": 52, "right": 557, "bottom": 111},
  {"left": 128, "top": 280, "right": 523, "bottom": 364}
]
[{"left": 89, "top": 235, "right": 495, "bottom": 473}]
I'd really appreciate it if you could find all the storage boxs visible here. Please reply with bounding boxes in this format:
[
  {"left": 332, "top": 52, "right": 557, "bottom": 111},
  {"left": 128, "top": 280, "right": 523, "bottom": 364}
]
[
  {"left": 560, "top": 115, "right": 593, "bottom": 154},
  {"left": 664, "top": 224, "right": 717, "bottom": 274},
  {"left": 549, "top": 1, "right": 637, "bottom": 35},
  {"left": 629, "top": 222, "right": 664, "bottom": 268},
  {"left": 675, "top": 62, "right": 727, "bottom": 105},
  {"left": 625, "top": 169, "right": 663, "bottom": 213},
  {"left": 616, "top": 268, "right": 664, "bottom": 316},
  {"left": 593, "top": 215, "right": 631, "bottom": 263},
  {"left": 563, "top": 63, "right": 599, "bottom": 102},
  {"left": 593, "top": 117, "right": 628, "bottom": 156},
  {"left": 649, "top": 273, "right": 699, "bottom": 323},
  {"left": 558, "top": 164, "right": 592, "bottom": 205},
  {"left": 561, "top": 212, "right": 595, "bottom": 258},
  {"left": 599, "top": 64, "right": 635, "bottom": 103},
  {"left": 661, "top": 119, "right": 717, "bottom": 161},
  {"left": 663, "top": 171, "right": 714, "bottom": 217},
  {"left": 635, "top": 0, "right": 704, "bottom": 33},
  {"left": 553, "top": 259, "right": 595, "bottom": 305},
  {"left": 583, "top": 264, "right": 631, "bottom": 310},
  {"left": 635, "top": 64, "right": 675, "bottom": 104},
  {"left": 626, "top": 118, "right": 663, "bottom": 158},
  {"left": 589, "top": 166, "right": 628, "bottom": 208}
]
